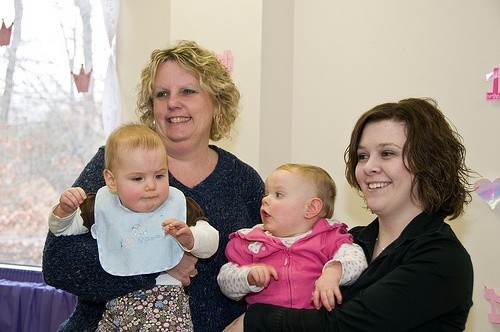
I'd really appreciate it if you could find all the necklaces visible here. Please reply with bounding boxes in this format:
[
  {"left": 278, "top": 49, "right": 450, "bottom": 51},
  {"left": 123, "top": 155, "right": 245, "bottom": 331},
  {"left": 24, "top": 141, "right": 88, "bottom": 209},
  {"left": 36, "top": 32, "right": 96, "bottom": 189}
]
[{"left": 376, "top": 237, "right": 384, "bottom": 251}]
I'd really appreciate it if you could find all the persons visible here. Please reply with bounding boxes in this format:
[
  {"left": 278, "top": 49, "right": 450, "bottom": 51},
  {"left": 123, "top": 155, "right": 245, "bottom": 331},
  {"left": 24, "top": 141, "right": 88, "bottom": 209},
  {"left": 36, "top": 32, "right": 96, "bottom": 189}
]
[
  {"left": 223, "top": 99, "right": 483, "bottom": 331},
  {"left": 47, "top": 124, "right": 219, "bottom": 332},
  {"left": 217, "top": 163, "right": 369, "bottom": 312},
  {"left": 41, "top": 40, "right": 266, "bottom": 332}
]
